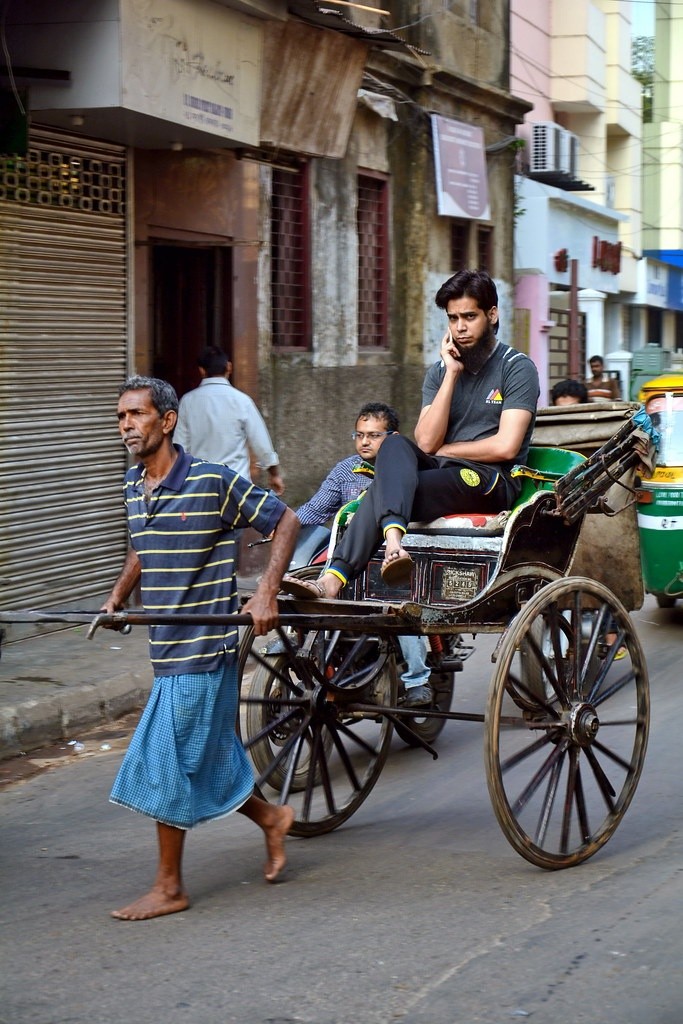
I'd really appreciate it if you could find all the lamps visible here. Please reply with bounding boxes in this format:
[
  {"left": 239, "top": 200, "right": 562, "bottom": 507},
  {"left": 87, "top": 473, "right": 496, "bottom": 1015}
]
[
  {"left": 69, "top": 115, "right": 85, "bottom": 125},
  {"left": 172, "top": 140, "right": 184, "bottom": 151}
]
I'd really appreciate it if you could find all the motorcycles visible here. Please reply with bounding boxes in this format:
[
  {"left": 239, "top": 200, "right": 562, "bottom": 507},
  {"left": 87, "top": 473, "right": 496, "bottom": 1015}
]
[
  {"left": 632, "top": 374, "right": 683, "bottom": 610},
  {"left": 245, "top": 524, "right": 476, "bottom": 795}
]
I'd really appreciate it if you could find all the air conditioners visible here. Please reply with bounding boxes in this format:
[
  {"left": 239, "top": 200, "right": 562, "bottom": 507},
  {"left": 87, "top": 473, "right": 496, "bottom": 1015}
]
[{"left": 530, "top": 120, "right": 582, "bottom": 175}]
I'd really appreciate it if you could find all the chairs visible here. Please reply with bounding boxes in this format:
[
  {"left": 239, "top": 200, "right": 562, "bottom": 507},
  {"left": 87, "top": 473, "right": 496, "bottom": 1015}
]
[{"left": 332, "top": 443, "right": 587, "bottom": 536}]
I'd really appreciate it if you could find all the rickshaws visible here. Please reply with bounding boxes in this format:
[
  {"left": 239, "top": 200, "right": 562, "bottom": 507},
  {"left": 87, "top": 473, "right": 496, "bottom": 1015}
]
[{"left": 0, "top": 402, "right": 661, "bottom": 872}]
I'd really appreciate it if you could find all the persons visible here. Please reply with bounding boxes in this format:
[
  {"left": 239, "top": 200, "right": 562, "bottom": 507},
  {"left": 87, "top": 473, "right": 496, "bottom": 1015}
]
[
  {"left": 170, "top": 344, "right": 286, "bottom": 497},
  {"left": 549, "top": 379, "right": 627, "bottom": 660},
  {"left": 583, "top": 355, "right": 624, "bottom": 404},
  {"left": 295, "top": 401, "right": 432, "bottom": 707},
  {"left": 275, "top": 269, "right": 541, "bottom": 599},
  {"left": 100, "top": 374, "right": 300, "bottom": 920}
]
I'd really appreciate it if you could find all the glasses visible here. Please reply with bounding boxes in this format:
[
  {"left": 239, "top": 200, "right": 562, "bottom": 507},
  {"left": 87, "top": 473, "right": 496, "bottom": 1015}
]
[{"left": 353, "top": 431, "right": 391, "bottom": 439}]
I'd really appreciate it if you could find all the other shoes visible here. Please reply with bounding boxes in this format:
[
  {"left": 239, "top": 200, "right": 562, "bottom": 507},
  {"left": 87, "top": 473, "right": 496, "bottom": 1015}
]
[{"left": 613, "top": 647, "right": 627, "bottom": 661}]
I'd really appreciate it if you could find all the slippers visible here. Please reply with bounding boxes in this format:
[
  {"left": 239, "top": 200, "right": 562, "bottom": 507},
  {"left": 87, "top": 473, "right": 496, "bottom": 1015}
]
[
  {"left": 280, "top": 576, "right": 337, "bottom": 599},
  {"left": 381, "top": 550, "right": 413, "bottom": 589}
]
